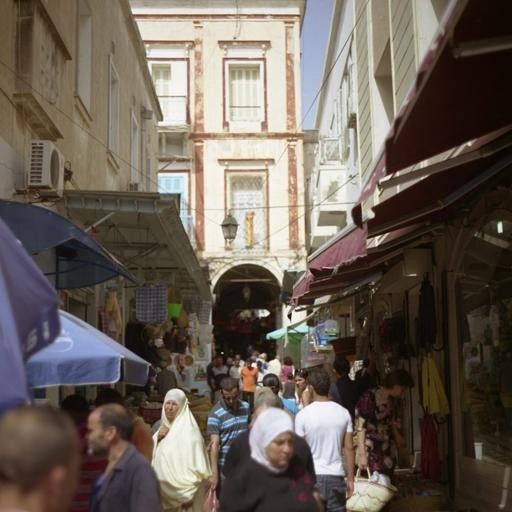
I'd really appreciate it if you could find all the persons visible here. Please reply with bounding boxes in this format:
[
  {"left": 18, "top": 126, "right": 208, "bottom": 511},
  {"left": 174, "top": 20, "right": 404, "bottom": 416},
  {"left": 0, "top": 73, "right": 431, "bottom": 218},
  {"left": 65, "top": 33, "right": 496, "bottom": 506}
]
[{"left": 0, "top": 344, "right": 417, "bottom": 511}]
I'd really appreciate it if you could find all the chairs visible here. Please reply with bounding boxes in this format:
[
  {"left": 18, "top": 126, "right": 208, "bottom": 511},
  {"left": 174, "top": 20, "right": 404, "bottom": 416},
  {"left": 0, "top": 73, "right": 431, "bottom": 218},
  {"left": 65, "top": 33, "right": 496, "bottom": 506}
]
[{"left": 393, "top": 451, "right": 426, "bottom": 502}]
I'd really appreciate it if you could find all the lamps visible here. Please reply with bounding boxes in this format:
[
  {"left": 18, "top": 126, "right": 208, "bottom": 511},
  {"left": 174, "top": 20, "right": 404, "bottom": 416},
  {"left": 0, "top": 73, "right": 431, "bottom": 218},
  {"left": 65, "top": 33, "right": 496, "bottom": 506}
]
[{"left": 221, "top": 212, "right": 239, "bottom": 245}]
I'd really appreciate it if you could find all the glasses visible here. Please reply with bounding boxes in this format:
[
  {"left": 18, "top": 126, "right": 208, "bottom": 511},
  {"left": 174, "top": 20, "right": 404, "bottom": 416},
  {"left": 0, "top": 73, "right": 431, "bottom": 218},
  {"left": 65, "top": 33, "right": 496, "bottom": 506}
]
[{"left": 223, "top": 395, "right": 237, "bottom": 400}]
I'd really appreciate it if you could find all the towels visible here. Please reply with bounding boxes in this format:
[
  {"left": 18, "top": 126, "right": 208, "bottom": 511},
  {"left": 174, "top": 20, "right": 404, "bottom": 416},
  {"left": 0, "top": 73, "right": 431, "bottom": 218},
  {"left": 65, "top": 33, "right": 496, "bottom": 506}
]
[{"left": 132, "top": 285, "right": 172, "bottom": 326}]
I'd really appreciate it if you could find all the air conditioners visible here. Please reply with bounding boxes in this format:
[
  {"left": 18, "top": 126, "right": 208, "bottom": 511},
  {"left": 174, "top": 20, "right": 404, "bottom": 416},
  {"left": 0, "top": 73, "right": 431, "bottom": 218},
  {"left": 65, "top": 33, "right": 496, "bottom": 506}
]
[{"left": 19, "top": 138, "right": 66, "bottom": 202}]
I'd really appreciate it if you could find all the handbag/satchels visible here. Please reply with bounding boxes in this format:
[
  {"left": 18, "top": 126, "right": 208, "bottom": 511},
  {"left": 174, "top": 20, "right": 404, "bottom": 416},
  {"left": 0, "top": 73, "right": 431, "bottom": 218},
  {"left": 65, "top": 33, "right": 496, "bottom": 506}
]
[
  {"left": 203, "top": 488, "right": 220, "bottom": 512},
  {"left": 344, "top": 466, "right": 398, "bottom": 511}
]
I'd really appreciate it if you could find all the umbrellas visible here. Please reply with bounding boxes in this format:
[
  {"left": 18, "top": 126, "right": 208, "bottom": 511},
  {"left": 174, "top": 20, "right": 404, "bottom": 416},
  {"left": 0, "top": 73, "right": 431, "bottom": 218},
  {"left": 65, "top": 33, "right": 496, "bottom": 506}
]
[{"left": 23, "top": 309, "right": 152, "bottom": 389}]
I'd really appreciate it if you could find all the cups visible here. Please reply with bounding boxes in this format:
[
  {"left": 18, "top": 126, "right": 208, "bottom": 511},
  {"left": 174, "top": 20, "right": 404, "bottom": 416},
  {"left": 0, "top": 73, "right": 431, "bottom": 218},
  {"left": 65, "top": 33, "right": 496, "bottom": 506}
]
[{"left": 473, "top": 442, "right": 484, "bottom": 461}]
